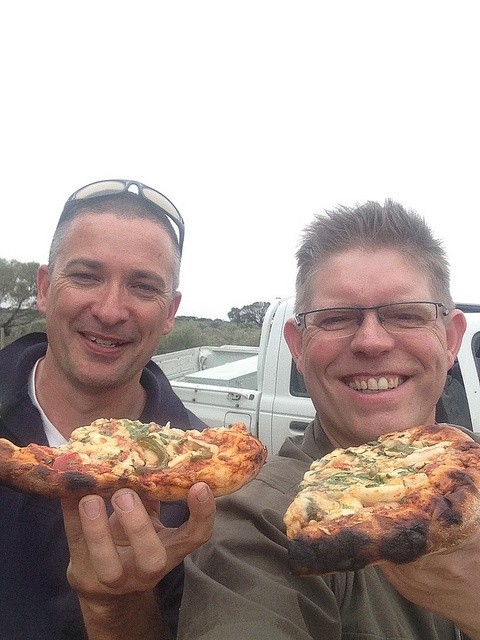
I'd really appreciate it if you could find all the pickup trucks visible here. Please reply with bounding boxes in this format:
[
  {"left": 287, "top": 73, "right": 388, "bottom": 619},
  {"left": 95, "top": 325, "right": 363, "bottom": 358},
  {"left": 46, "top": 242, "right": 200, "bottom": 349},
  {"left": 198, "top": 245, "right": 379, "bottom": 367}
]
[{"left": 151, "top": 288, "right": 480, "bottom": 457}]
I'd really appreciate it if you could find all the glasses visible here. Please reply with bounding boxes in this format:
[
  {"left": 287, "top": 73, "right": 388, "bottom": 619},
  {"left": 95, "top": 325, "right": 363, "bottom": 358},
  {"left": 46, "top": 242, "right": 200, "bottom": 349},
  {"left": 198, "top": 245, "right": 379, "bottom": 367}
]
[
  {"left": 295, "top": 301, "right": 449, "bottom": 339},
  {"left": 56, "top": 179, "right": 185, "bottom": 257}
]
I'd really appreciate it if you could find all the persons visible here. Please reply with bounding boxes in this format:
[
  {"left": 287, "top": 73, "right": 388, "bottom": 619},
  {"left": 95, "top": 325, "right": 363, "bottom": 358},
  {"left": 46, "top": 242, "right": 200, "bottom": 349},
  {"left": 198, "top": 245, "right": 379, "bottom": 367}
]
[
  {"left": 0, "top": 177, "right": 217, "bottom": 640},
  {"left": 178, "top": 199, "right": 480, "bottom": 639}
]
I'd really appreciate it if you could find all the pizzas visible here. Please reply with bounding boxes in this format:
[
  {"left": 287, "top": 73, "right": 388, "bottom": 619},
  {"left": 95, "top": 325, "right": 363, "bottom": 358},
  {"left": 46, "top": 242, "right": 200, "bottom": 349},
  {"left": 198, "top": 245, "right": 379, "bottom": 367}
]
[
  {"left": 283, "top": 423, "right": 480, "bottom": 576},
  {"left": 1, "top": 418, "right": 267, "bottom": 504}
]
